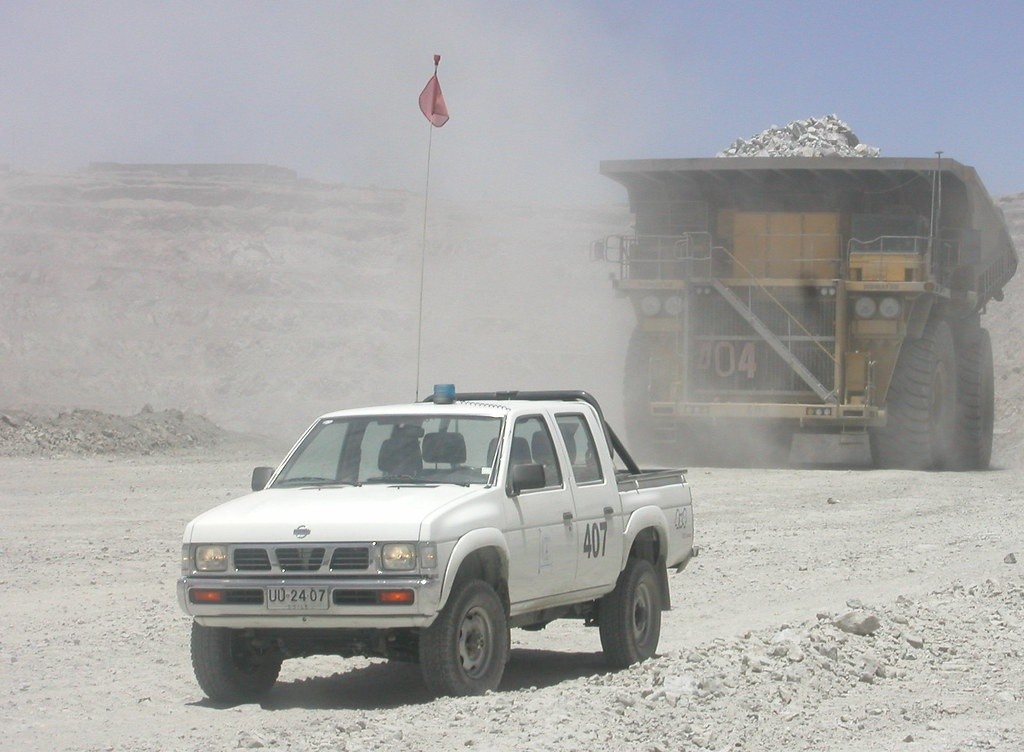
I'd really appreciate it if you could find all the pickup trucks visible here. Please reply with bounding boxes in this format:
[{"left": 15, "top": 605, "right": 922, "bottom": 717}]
[{"left": 174, "top": 381, "right": 701, "bottom": 704}]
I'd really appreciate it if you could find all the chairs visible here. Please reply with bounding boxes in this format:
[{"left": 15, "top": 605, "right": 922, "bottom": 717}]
[
  {"left": 366, "top": 439, "right": 423, "bottom": 481},
  {"left": 531, "top": 431, "right": 581, "bottom": 485},
  {"left": 421, "top": 431, "right": 467, "bottom": 474},
  {"left": 486, "top": 437, "right": 532, "bottom": 474}
]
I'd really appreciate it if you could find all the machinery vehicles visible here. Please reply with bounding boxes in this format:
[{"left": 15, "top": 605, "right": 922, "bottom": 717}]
[{"left": 596, "top": 154, "right": 1019, "bottom": 469}]
